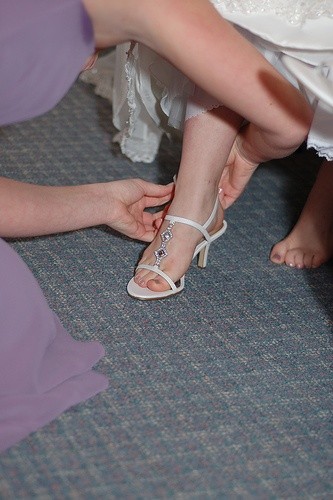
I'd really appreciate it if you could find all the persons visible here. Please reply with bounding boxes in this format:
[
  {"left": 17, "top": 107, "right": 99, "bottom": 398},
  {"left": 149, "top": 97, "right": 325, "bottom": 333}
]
[
  {"left": 0, "top": 0, "right": 314, "bottom": 456},
  {"left": 126, "top": 0, "right": 333, "bottom": 301}
]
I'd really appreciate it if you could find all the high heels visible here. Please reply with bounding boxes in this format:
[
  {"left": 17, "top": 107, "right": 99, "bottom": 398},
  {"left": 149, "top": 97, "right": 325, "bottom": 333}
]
[{"left": 126, "top": 187, "right": 227, "bottom": 300}]
